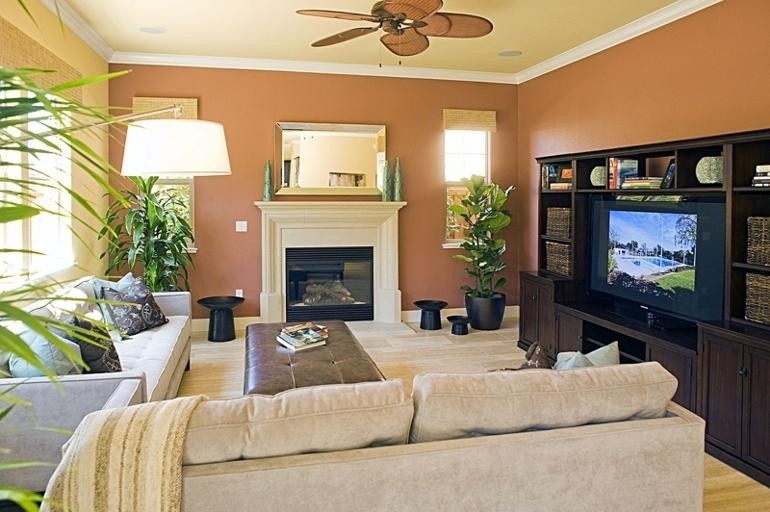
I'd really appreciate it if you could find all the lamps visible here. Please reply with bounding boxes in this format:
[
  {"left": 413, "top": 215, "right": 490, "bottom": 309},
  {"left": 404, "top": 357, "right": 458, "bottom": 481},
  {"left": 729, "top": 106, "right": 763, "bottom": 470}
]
[{"left": 1, "top": 105, "right": 232, "bottom": 178}]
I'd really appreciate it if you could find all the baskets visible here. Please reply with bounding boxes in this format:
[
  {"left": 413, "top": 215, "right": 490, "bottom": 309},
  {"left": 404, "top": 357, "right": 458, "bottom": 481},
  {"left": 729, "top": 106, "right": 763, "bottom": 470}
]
[
  {"left": 546, "top": 207, "right": 572, "bottom": 239},
  {"left": 746, "top": 215, "right": 770, "bottom": 268},
  {"left": 546, "top": 241, "right": 572, "bottom": 275},
  {"left": 744, "top": 272, "right": 769, "bottom": 326}
]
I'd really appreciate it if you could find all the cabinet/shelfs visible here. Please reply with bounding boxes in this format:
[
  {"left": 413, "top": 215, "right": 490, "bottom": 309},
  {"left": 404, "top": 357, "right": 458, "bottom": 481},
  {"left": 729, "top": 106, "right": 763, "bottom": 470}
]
[
  {"left": 556, "top": 300, "right": 696, "bottom": 414},
  {"left": 725, "top": 127, "right": 769, "bottom": 330},
  {"left": 517, "top": 271, "right": 587, "bottom": 367},
  {"left": 535, "top": 151, "right": 587, "bottom": 279},
  {"left": 575, "top": 133, "right": 724, "bottom": 193},
  {"left": 696, "top": 319, "right": 770, "bottom": 487}
]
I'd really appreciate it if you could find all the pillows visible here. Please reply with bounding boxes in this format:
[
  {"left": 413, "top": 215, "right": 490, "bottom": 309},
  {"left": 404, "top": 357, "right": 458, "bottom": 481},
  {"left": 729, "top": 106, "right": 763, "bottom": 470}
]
[{"left": 0, "top": 272, "right": 168, "bottom": 380}]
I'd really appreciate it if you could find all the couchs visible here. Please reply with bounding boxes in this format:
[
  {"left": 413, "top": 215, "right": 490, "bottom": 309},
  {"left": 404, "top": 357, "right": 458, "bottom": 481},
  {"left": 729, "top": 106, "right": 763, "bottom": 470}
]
[
  {"left": 0, "top": 275, "right": 192, "bottom": 494},
  {"left": 83, "top": 361, "right": 706, "bottom": 511}
]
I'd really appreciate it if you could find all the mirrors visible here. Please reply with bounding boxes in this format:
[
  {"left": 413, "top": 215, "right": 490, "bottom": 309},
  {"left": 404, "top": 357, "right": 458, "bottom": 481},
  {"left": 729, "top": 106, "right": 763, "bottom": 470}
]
[{"left": 274, "top": 121, "right": 389, "bottom": 198}]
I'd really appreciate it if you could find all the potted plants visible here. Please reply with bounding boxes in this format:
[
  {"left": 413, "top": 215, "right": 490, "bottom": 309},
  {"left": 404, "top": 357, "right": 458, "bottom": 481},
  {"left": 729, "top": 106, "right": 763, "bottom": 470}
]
[{"left": 448, "top": 174, "right": 517, "bottom": 331}]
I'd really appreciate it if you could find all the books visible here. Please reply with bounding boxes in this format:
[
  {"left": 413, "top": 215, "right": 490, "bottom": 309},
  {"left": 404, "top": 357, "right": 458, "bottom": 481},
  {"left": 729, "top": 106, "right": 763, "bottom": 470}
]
[
  {"left": 542, "top": 162, "right": 573, "bottom": 190},
  {"left": 752, "top": 165, "right": 770, "bottom": 187},
  {"left": 276, "top": 321, "right": 328, "bottom": 351},
  {"left": 660, "top": 158, "right": 675, "bottom": 190},
  {"left": 621, "top": 175, "right": 664, "bottom": 189},
  {"left": 609, "top": 157, "right": 638, "bottom": 190}
]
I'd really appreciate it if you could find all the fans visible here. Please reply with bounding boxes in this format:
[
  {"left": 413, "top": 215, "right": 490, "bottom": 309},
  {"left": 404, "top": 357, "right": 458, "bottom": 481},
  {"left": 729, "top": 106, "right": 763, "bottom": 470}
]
[{"left": 296, "top": 0, "right": 493, "bottom": 68}]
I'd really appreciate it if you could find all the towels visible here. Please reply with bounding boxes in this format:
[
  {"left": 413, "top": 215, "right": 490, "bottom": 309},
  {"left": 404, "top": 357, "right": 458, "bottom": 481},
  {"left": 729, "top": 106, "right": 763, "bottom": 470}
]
[{"left": 39, "top": 394, "right": 208, "bottom": 511}]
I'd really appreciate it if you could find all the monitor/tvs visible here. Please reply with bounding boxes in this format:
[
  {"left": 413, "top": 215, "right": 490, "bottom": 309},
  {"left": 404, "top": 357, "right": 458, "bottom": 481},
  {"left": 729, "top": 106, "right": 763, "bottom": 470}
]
[{"left": 585, "top": 194, "right": 726, "bottom": 330}]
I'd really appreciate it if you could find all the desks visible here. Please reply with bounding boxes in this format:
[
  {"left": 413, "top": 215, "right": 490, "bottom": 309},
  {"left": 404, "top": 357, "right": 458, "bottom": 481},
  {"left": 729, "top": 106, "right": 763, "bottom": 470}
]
[{"left": 243, "top": 320, "right": 387, "bottom": 396}]
